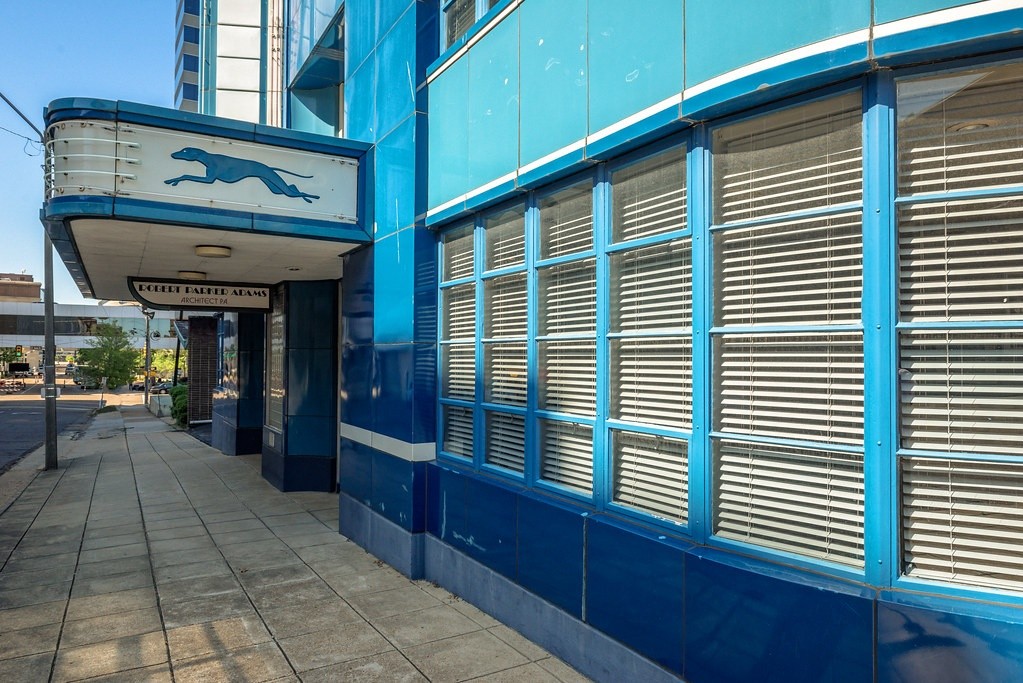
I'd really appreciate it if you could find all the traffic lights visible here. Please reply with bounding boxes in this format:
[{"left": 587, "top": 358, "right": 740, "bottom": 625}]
[{"left": 16, "top": 345, "right": 22, "bottom": 357}]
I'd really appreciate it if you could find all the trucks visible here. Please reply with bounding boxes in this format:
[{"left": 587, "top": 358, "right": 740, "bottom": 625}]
[{"left": 73, "top": 365, "right": 99, "bottom": 390}]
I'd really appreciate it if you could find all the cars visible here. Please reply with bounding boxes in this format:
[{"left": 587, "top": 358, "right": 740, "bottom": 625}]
[
  {"left": 150, "top": 382, "right": 180, "bottom": 394},
  {"left": 37, "top": 367, "right": 43, "bottom": 375},
  {"left": 65, "top": 364, "right": 76, "bottom": 375}
]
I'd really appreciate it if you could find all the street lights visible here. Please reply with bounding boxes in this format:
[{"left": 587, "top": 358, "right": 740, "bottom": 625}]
[{"left": 119, "top": 301, "right": 150, "bottom": 404}]
